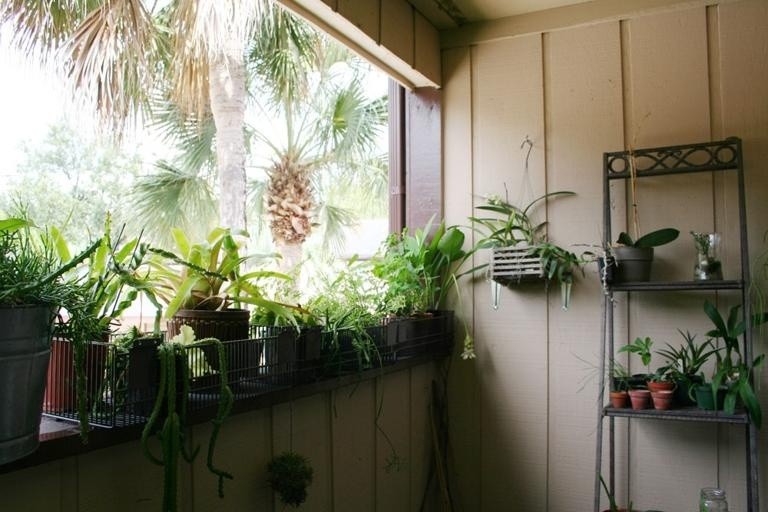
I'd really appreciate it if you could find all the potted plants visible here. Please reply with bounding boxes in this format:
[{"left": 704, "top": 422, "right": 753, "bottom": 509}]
[
  {"left": 107, "top": 324, "right": 189, "bottom": 420},
  {"left": 1, "top": 213, "right": 104, "bottom": 466},
  {"left": 607, "top": 300, "right": 758, "bottom": 416},
  {"left": 163, "top": 224, "right": 288, "bottom": 394},
  {"left": 470, "top": 192, "right": 590, "bottom": 284},
  {"left": 603, "top": 225, "right": 724, "bottom": 284},
  {"left": 370, "top": 211, "right": 468, "bottom": 360},
  {"left": 1, "top": 208, "right": 467, "bottom": 510},
  {"left": 330, "top": 256, "right": 389, "bottom": 367},
  {"left": 41, "top": 220, "right": 139, "bottom": 421},
  {"left": 249, "top": 294, "right": 323, "bottom": 370}
]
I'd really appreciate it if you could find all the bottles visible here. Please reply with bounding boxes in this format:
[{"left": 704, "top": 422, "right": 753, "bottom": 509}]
[{"left": 699, "top": 487, "right": 728, "bottom": 512}]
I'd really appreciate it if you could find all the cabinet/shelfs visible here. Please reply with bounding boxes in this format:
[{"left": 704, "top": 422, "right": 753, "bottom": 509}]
[{"left": 593, "top": 133, "right": 760, "bottom": 510}]
